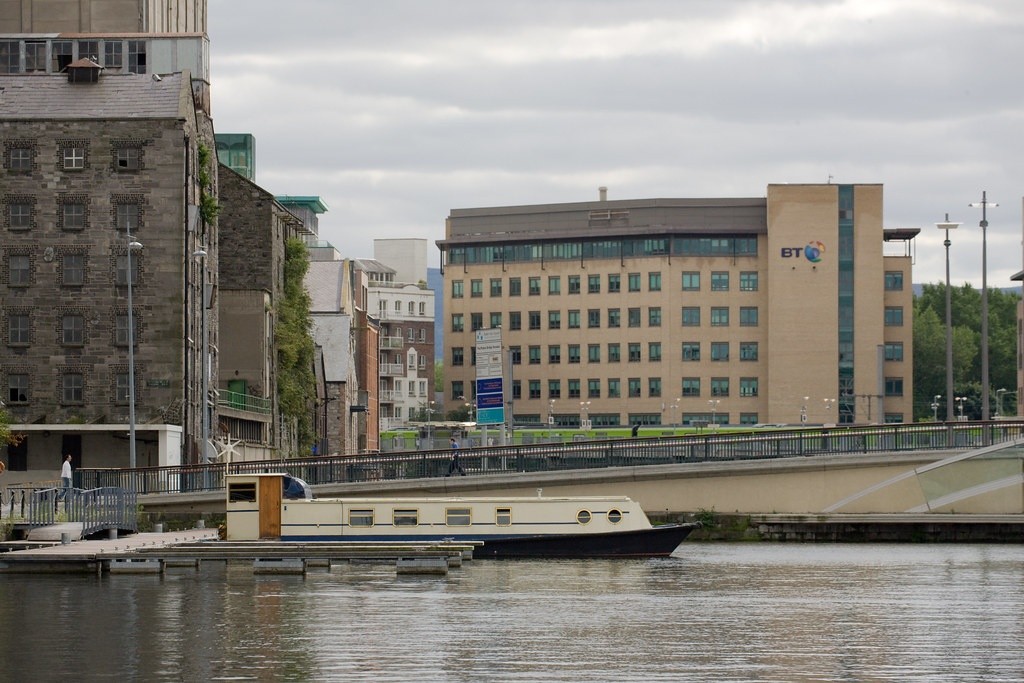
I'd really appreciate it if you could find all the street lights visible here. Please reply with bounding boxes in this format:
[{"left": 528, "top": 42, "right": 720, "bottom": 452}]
[
  {"left": 994, "top": 389, "right": 1006, "bottom": 420},
  {"left": 709, "top": 399, "right": 720, "bottom": 430},
  {"left": 967, "top": 190, "right": 999, "bottom": 450},
  {"left": 580, "top": 400, "right": 591, "bottom": 428},
  {"left": 932, "top": 395, "right": 942, "bottom": 423},
  {"left": 801, "top": 396, "right": 809, "bottom": 427},
  {"left": 465, "top": 401, "right": 476, "bottom": 448},
  {"left": 548, "top": 400, "right": 555, "bottom": 430},
  {"left": 130, "top": 221, "right": 143, "bottom": 469},
  {"left": 935, "top": 212, "right": 965, "bottom": 446},
  {"left": 824, "top": 398, "right": 835, "bottom": 426},
  {"left": 427, "top": 401, "right": 434, "bottom": 451},
  {"left": 956, "top": 397, "right": 968, "bottom": 421},
  {"left": 672, "top": 397, "right": 680, "bottom": 436},
  {"left": 192, "top": 249, "right": 210, "bottom": 490}
]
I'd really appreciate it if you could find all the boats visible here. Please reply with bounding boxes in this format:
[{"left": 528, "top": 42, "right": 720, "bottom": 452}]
[{"left": 193, "top": 466, "right": 703, "bottom": 561}]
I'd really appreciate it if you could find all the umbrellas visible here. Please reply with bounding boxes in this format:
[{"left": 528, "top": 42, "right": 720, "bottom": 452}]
[{"left": 632, "top": 425, "right": 639, "bottom": 430}]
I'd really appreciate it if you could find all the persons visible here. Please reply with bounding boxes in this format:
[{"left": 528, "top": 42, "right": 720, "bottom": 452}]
[
  {"left": 632, "top": 428, "right": 637, "bottom": 437},
  {"left": 59, "top": 455, "right": 72, "bottom": 501},
  {"left": 445, "top": 439, "right": 466, "bottom": 476}
]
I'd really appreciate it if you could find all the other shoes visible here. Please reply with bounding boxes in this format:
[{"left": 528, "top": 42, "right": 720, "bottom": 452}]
[{"left": 59, "top": 495, "right": 62, "bottom": 501}]
